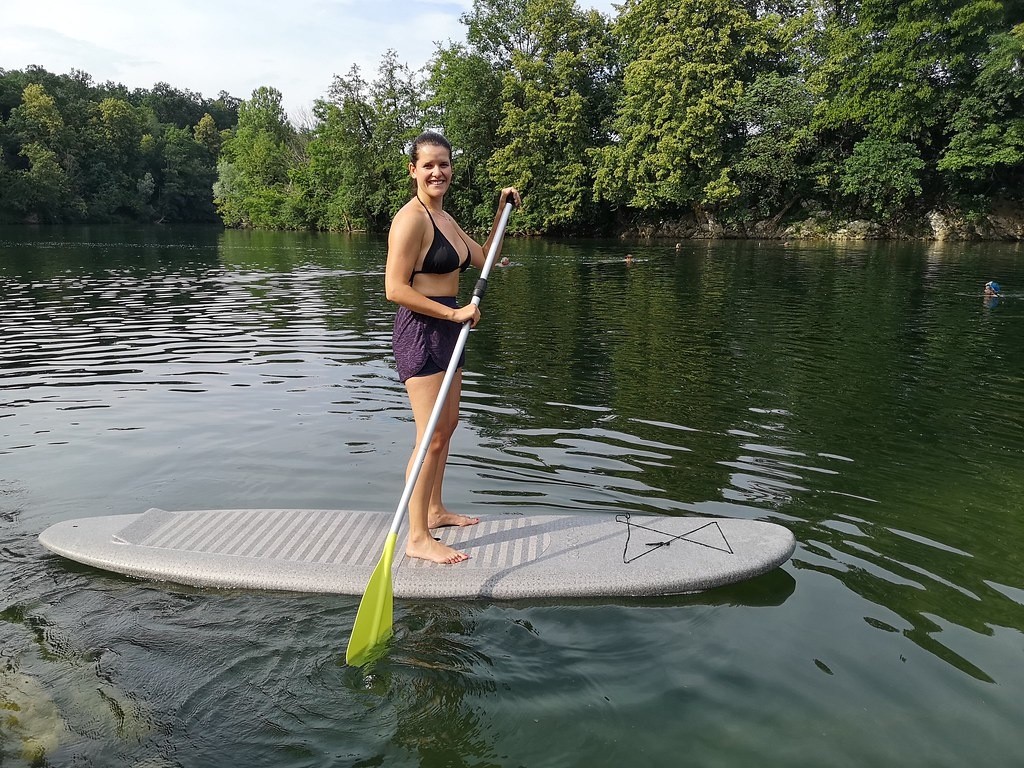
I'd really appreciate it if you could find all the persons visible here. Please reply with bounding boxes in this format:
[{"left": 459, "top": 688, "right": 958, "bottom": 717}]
[
  {"left": 984, "top": 281, "right": 1000, "bottom": 295},
  {"left": 385, "top": 131, "right": 521, "bottom": 564}
]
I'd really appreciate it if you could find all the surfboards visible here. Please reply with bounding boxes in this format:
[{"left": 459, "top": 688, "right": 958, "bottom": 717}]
[{"left": 34, "top": 507, "right": 797, "bottom": 602}]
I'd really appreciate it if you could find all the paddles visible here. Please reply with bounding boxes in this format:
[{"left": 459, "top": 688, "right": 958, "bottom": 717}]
[{"left": 344, "top": 193, "right": 517, "bottom": 664}]
